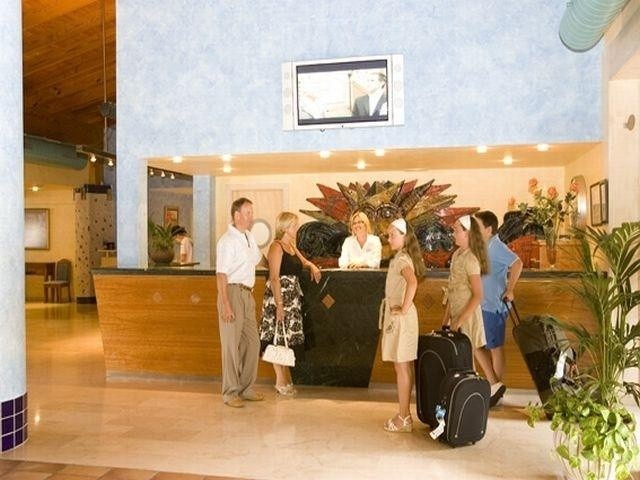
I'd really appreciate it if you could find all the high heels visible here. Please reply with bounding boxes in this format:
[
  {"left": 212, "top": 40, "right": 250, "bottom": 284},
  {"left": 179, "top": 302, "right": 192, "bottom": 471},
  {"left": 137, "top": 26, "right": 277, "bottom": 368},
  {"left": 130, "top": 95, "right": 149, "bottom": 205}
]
[{"left": 383, "top": 413, "right": 412, "bottom": 432}]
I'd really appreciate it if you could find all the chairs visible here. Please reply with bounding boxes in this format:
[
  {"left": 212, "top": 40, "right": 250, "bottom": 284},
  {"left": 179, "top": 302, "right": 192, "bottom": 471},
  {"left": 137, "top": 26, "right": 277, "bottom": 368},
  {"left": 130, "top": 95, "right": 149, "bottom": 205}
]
[{"left": 43, "top": 258, "right": 72, "bottom": 304}]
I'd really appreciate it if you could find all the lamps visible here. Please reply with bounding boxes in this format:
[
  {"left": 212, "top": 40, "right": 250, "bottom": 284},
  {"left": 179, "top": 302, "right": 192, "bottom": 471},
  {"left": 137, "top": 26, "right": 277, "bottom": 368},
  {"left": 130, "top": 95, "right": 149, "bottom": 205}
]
[{"left": 75, "top": 144, "right": 178, "bottom": 181}]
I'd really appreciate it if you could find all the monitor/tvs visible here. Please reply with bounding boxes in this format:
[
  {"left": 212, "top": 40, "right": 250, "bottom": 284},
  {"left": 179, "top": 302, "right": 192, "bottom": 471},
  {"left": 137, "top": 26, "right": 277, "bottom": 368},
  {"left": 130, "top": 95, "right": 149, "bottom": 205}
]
[{"left": 281, "top": 53, "right": 405, "bottom": 132}]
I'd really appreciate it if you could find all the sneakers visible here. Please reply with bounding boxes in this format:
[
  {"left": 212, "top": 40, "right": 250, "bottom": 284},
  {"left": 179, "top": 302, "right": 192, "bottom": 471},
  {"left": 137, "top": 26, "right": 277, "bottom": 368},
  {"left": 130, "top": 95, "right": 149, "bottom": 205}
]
[
  {"left": 246, "top": 391, "right": 265, "bottom": 400},
  {"left": 228, "top": 398, "right": 244, "bottom": 407},
  {"left": 490, "top": 380, "right": 506, "bottom": 408}
]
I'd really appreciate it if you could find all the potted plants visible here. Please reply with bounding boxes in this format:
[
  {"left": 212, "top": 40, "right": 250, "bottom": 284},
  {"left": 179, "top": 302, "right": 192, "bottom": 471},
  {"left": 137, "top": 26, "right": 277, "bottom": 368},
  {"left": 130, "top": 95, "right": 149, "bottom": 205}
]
[
  {"left": 148, "top": 215, "right": 186, "bottom": 263},
  {"left": 515, "top": 218, "right": 640, "bottom": 480}
]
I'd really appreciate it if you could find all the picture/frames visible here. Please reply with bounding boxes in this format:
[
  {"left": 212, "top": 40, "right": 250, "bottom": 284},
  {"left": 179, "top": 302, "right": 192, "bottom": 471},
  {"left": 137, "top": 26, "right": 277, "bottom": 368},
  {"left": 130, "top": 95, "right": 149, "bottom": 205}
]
[
  {"left": 589, "top": 180, "right": 601, "bottom": 226},
  {"left": 600, "top": 180, "right": 609, "bottom": 224},
  {"left": 164, "top": 205, "right": 181, "bottom": 226},
  {"left": 25, "top": 207, "right": 51, "bottom": 251}
]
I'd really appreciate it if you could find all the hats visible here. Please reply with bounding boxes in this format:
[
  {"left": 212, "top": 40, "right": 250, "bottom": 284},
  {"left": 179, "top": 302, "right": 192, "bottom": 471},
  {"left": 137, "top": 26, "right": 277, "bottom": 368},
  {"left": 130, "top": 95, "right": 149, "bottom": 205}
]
[{"left": 171, "top": 225, "right": 184, "bottom": 236}]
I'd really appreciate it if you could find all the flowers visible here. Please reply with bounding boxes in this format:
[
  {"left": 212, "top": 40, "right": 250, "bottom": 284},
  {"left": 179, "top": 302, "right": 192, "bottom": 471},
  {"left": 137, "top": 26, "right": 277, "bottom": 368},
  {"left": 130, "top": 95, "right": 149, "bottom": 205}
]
[{"left": 505, "top": 176, "right": 584, "bottom": 242}]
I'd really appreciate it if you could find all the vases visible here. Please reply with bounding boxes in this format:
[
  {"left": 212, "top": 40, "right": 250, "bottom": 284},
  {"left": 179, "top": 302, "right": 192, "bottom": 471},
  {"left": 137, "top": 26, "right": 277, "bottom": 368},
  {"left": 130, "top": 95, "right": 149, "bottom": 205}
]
[{"left": 530, "top": 237, "right": 549, "bottom": 269}]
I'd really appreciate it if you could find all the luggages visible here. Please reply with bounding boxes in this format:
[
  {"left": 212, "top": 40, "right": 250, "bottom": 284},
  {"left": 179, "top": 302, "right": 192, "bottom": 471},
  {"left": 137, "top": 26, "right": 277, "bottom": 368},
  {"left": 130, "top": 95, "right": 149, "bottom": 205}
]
[
  {"left": 414, "top": 325, "right": 472, "bottom": 429},
  {"left": 504, "top": 297, "right": 580, "bottom": 421},
  {"left": 439, "top": 369, "right": 491, "bottom": 448}
]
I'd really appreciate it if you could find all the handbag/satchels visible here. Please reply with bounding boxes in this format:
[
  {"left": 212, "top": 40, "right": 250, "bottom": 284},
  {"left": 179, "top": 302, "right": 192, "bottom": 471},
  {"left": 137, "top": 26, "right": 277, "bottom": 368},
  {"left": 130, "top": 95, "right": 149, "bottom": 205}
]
[{"left": 263, "top": 344, "right": 295, "bottom": 367}]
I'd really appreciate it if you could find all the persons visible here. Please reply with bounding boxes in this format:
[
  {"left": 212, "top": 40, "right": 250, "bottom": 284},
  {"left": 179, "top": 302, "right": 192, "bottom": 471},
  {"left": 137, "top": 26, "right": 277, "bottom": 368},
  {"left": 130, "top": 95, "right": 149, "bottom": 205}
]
[
  {"left": 171, "top": 225, "right": 193, "bottom": 265},
  {"left": 377, "top": 217, "right": 426, "bottom": 433},
  {"left": 258, "top": 211, "right": 322, "bottom": 396},
  {"left": 350, "top": 72, "right": 386, "bottom": 117},
  {"left": 214, "top": 198, "right": 269, "bottom": 408},
  {"left": 474, "top": 209, "right": 524, "bottom": 380},
  {"left": 441, "top": 213, "right": 508, "bottom": 408},
  {"left": 337, "top": 209, "right": 383, "bottom": 269}
]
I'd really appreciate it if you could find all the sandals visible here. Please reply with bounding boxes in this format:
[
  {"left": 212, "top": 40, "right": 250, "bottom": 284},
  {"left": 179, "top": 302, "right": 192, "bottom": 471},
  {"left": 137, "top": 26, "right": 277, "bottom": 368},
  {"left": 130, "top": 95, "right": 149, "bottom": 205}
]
[{"left": 274, "top": 381, "right": 298, "bottom": 396}]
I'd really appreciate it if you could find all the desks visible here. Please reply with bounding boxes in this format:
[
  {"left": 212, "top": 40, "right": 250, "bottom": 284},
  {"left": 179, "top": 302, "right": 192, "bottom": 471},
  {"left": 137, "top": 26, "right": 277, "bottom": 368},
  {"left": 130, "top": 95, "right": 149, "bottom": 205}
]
[
  {"left": 25, "top": 261, "right": 55, "bottom": 304},
  {"left": 98, "top": 249, "right": 116, "bottom": 268}
]
